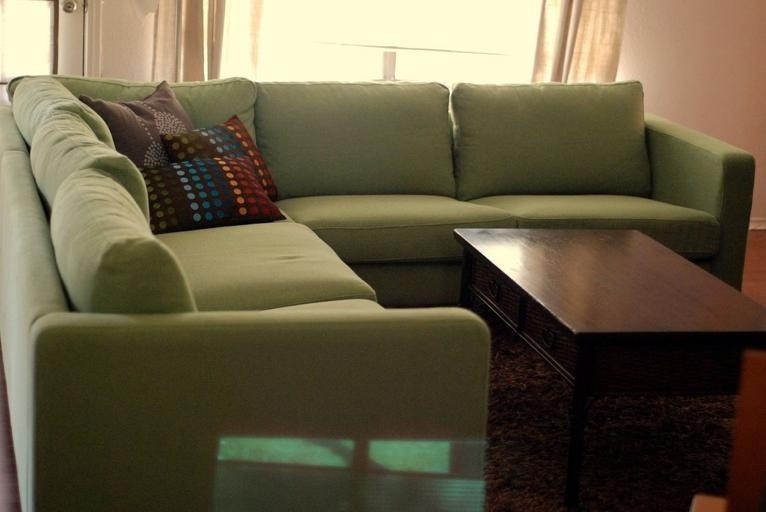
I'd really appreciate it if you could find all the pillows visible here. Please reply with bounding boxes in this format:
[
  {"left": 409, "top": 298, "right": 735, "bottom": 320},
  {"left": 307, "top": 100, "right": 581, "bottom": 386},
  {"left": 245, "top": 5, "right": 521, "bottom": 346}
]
[
  {"left": 139, "top": 153, "right": 287, "bottom": 238},
  {"left": 78, "top": 80, "right": 193, "bottom": 169},
  {"left": 158, "top": 116, "right": 279, "bottom": 205}
]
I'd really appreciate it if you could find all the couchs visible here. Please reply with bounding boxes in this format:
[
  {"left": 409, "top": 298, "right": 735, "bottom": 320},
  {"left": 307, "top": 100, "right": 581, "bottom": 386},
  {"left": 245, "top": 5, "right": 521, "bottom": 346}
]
[{"left": 0, "top": 78, "right": 756, "bottom": 512}]
